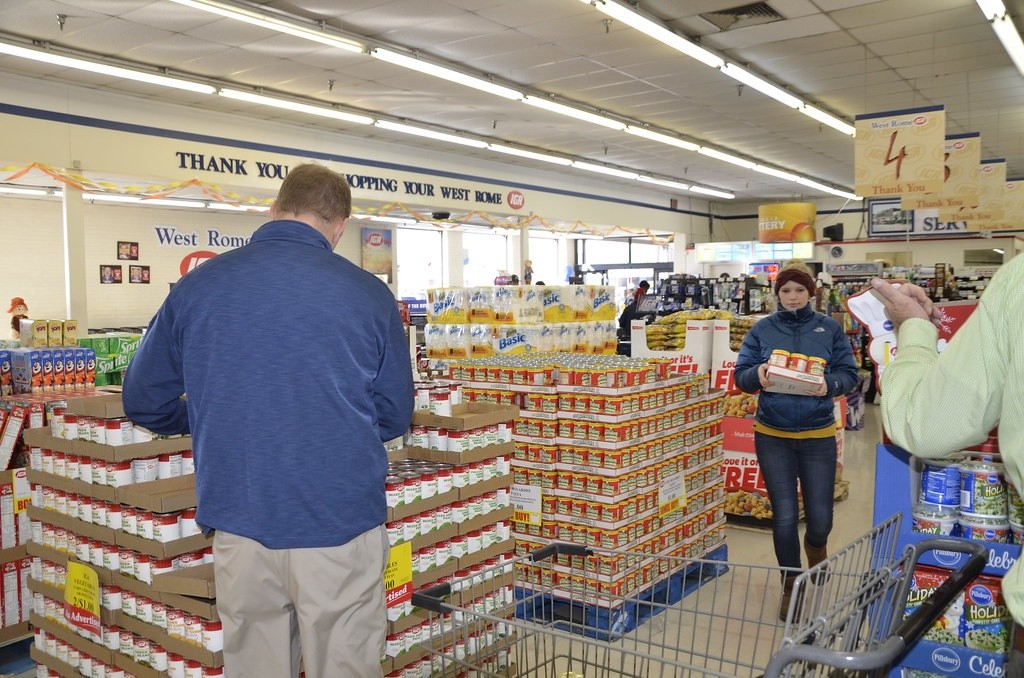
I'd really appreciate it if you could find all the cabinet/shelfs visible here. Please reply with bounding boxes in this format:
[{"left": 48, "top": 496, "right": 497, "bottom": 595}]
[{"left": 638, "top": 266, "right": 992, "bottom": 429}]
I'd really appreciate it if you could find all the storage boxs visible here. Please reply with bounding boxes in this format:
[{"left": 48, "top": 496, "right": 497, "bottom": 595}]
[{"left": 0, "top": 331, "right": 519, "bottom": 678}]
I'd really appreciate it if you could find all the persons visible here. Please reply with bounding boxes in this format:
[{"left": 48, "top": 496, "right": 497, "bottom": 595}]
[
  {"left": 122, "top": 164, "right": 415, "bottom": 678},
  {"left": 733, "top": 258, "right": 860, "bottom": 624},
  {"left": 870, "top": 250, "right": 1024, "bottom": 628},
  {"left": 102, "top": 267, "right": 113, "bottom": 281},
  {"left": 119, "top": 244, "right": 129, "bottom": 257},
  {"left": 625, "top": 280, "right": 650, "bottom": 307}
]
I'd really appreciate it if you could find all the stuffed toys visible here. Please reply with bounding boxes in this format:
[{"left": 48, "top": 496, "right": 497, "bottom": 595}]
[{"left": 6, "top": 297, "right": 29, "bottom": 337}]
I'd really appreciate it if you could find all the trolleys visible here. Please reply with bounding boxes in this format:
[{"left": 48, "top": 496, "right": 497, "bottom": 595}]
[{"left": 409, "top": 508, "right": 990, "bottom": 677}]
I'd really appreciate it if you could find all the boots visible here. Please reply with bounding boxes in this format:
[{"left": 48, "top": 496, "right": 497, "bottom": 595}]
[
  {"left": 778, "top": 574, "right": 806, "bottom": 622},
  {"left": 803, "top": 532, "right": 832, "bottom": 585}
]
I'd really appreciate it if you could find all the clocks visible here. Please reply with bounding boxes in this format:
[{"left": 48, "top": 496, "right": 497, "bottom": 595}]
[{"left": 830, "top": 246, "right": 844, "bottom": 257}]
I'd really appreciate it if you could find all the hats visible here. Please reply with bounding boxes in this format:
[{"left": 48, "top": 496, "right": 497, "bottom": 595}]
[{"left": 508, "top": 275, "right": 520, "bottom": 282}]
